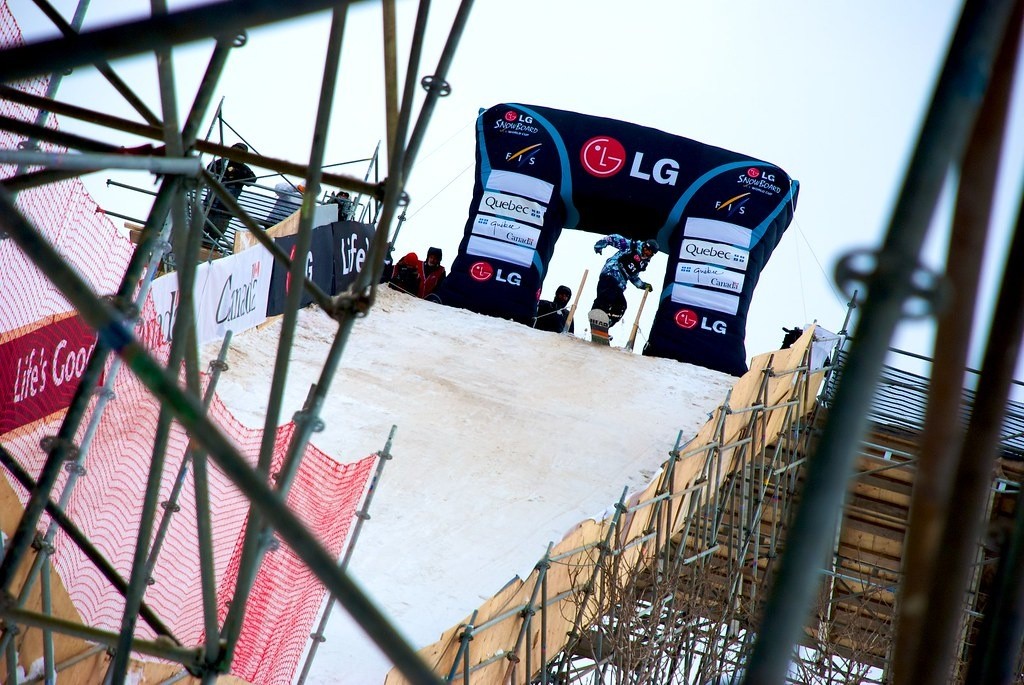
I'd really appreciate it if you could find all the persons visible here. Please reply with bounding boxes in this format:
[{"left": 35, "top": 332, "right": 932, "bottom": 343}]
[
  {"left": 200, "top": 143, "right": 257, "bottom": 251},
  {"left": 379, "top": 247, "right": 447, "bottom": 302},
  {"left": 264, "top": 180, "right": 351, "bottom": 238},
  {"left": 534, "top": 285, "right": 574, "bottom": 335},
  {"left": 591, "top": 234, "right": 660, "bottom": 341}
]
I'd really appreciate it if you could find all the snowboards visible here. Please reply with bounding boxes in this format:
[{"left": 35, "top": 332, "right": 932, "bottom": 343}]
[{"left": 588, "top": 308, "right": 610, "bottom": 345}]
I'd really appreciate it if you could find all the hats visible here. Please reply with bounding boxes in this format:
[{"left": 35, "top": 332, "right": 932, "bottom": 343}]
[
  {"left": 556, "top": 285, "right": 572, "bottom": 297},
  {"left": 427, "top": 247, "right": 443, "bottom": 261},
  {"left": 644, "top": 240, "right": 660, "bottom": 254}
]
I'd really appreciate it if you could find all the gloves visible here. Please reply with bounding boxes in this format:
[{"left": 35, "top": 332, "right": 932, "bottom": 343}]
[
  {"left": 595, "top": 250, "right": 603, "bottom": 255},
  {"left": 643, "top": 283, "right": 653, "bottom": 292},
  {"left": 298, "top": 186, "right": 305, "bottom": 191}
]
